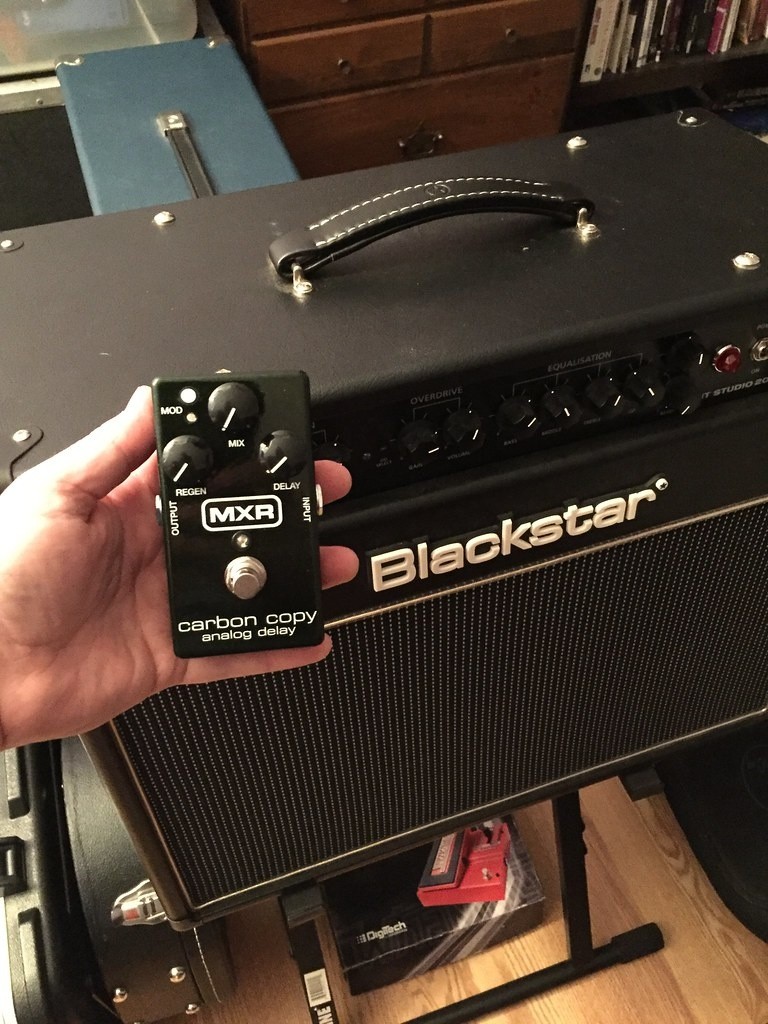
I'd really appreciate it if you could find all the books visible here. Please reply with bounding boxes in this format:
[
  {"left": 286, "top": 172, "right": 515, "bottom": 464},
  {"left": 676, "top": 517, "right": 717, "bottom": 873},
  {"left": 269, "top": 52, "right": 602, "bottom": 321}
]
[{"left": 579, "top": 0, "right": 768, "bottom": 83}]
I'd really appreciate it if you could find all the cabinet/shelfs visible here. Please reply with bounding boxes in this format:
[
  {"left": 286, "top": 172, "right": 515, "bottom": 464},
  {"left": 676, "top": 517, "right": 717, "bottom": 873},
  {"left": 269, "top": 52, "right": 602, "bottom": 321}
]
[{"left": 211, "top": 1, "right": 583, "bottom": 178}]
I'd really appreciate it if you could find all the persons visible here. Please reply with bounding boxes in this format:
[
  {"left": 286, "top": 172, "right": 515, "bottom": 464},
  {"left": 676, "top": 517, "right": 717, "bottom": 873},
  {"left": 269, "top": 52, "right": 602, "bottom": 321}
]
[{"left": 0, "top": 384, "right": 360, "bottom": 753}]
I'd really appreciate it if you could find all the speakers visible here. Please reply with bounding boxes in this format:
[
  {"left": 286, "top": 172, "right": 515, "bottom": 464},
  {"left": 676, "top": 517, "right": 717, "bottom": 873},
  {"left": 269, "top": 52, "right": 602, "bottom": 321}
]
[{"left": 0, "top": 108, "right": 768, "bottom": 932}]
